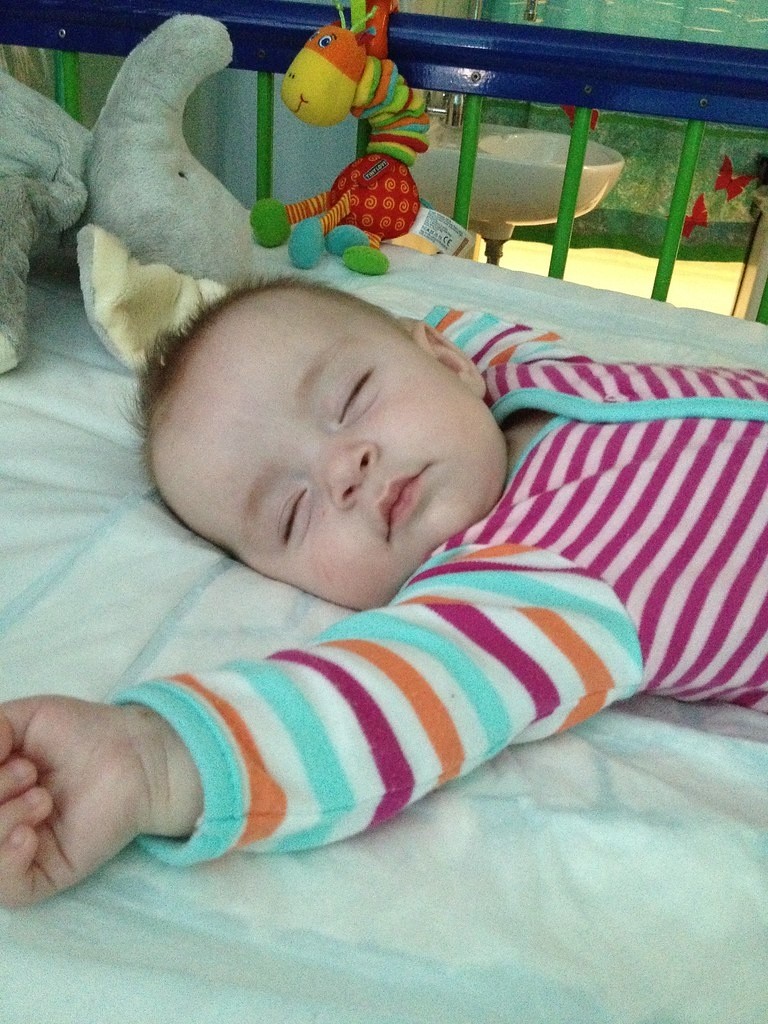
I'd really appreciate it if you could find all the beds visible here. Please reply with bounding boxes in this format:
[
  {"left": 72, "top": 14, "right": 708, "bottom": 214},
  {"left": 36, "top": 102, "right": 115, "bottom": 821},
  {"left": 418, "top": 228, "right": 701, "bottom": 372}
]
[{"left": 0, "top": 0, "right": 768, "bottom": 1024}]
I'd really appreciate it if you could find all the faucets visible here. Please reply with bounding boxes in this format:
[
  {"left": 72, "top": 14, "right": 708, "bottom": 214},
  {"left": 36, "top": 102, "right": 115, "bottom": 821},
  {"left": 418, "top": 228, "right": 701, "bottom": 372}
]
[
  {"left": 522, "top": 0, "right": 537, "bottom": 23},
  {"left": 438, "top": 90, "right": 465, "bottom": 129}
]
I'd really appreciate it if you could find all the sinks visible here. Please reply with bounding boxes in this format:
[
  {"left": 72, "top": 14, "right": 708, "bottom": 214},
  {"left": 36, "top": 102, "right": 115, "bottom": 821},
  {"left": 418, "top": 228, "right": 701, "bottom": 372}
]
[{"left": 427, "top": 124, "right": 626, "bottom": 227}]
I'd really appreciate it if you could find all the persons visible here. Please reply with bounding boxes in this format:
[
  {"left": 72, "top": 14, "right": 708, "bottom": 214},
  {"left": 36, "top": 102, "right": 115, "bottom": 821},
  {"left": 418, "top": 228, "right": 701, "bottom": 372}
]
[{"left": 0, "top": 275, "right": 768, "bottom": 911}]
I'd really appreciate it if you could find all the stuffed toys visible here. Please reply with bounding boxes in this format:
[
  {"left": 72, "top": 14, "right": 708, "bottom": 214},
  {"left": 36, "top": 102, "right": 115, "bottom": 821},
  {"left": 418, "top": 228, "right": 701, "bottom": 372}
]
[
  {"left": 248, "top": 4, "right": 430, "bottom": 272},
  {"left": 0, "top": 14, "right": 253, "bottom": 372}
]
